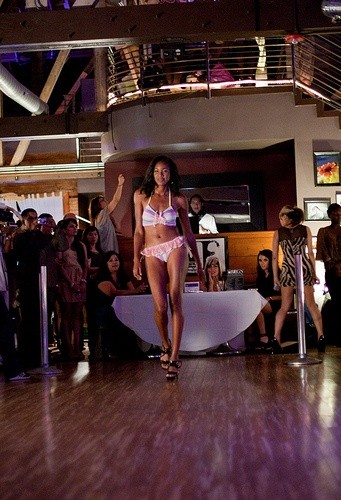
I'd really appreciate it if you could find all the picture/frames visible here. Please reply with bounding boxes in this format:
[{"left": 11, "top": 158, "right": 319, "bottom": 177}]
[
  {"left": 313, "top": 151, "right": 341, "bottom": 187},
  {"left": 303, "top": 197, "right": 331, "bottom": 222},
  {"left": 186, "top": 236, "right": 228, "bottom": 275}
]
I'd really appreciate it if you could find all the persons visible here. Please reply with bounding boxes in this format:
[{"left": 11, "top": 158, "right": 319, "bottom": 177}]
[
  {"left": 316, "top": 203, "right": 341, "bottom": 347},
  {"left": 133, "top": 155, "right": 206, "bottom": 381},
  {"left": 269, "top": 206, "right": 326, "bottom": 355},
  {"left": 244, "top": 249, "right": 282, "bottom": 353},
  {"left": 200, "top": 256, "right": 226, "bottom": 292},
  {"left": 188, "top": 194, "right": 218, "bottom": 235},
  {"left": 0, "top": 174, "right": 149, "bottom": 381},
  {"left": 143, "top": 53, "right": 257, "bottom": 89}
]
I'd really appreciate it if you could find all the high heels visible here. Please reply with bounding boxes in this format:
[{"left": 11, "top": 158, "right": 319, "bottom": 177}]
[
  {"left": 160, "top": 339, "right": 173, "bottom": 370},
  {"left": 166, "top": 357, "right": 180, "bottom": 382},
  {"left": 269, "top": 336, "right": 282, "bottom": 353},
  {"left": 316, "top": 335, "right": 326, "bottom": 355}
]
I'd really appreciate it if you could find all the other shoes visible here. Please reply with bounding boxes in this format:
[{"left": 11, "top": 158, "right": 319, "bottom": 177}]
[
  {"left": 9, "top": 371, "right": 32, "bottom": 381},
  {"left": 254, "top": 333, "right": 270, "bottom": 352},
  {"left": 247, "top": 332, "right": 254, "bottom": 346}
]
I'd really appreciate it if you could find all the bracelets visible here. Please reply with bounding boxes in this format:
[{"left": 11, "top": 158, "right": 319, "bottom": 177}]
[
  {"left": 195, "top": 260, "right": 200, "bottom": 264},
  {"left": 81, "top": 279, "right": 87, "bottom": 283},
  {"left": 71, "top": 280, "right": 74, "bottom": 288},
  {"left": 268, "top": 296, "right": 272, "bottom": 302},
  {"left": 117, "top": 184, "right": 124, "bottom": 186}
]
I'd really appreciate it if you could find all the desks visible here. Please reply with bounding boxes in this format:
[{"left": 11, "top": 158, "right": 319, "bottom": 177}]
[{"left": 112, "top": 288, "right": 269, "bottom": 357}]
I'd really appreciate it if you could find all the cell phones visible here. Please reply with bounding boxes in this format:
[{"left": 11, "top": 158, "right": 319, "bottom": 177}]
[{"left": 36, "top": 218, "right": 46, "bottom": 224}]
